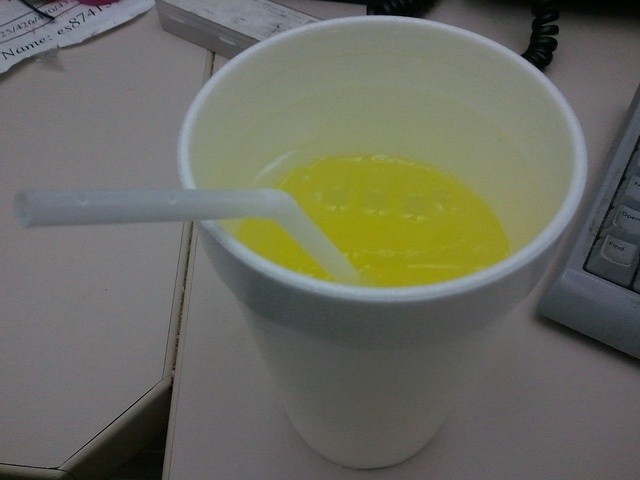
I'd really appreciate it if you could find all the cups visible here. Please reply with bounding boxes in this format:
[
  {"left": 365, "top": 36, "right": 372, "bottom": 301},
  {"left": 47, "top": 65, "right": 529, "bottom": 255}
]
[{"left": 175, "top": 17, "right": 586, "bottom": 470}]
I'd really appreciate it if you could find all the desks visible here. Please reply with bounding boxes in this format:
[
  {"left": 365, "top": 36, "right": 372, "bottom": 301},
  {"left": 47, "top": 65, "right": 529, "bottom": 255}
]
[
  {"left": 0, "top": 0, "right": 215, "bottom": 479},
  {"left": 162, "top": 1, "right": 640, "bottom": 477}
]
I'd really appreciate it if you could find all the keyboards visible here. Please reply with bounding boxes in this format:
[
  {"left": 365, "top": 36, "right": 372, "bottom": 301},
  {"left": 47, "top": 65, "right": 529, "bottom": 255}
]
[{"left": 534, "top": 72, "right": 640, "bottom": 362}]
